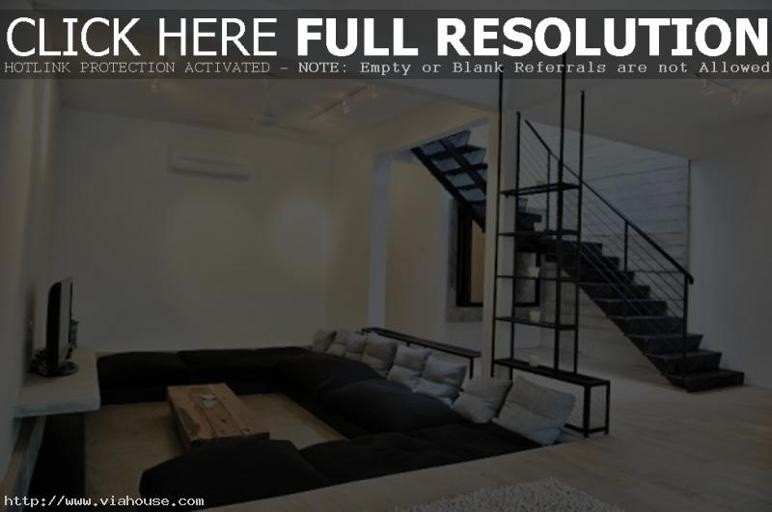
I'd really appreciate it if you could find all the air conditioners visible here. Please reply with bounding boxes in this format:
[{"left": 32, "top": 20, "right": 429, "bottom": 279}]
[{"left": 167, "top": 142, "right": 251, "bottom": 179}]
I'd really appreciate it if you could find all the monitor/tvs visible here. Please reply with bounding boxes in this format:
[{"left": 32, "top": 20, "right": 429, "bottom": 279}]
[{"left": 28, "top": 276, "right": 80, "bottom": 379}]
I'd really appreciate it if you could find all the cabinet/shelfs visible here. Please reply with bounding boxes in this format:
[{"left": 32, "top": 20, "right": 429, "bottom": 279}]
[{"left": 487, "top": 58, "right": 610, "bottom": 441}]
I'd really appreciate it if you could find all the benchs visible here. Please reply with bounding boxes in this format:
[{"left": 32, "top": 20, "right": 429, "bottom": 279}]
[{"left": 364, "top": 324, "right": 482, "bottom": 383}]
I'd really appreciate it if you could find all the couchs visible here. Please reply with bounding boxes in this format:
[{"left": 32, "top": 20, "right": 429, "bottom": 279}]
[{"left": 98, "top": 347, "right": 559, "bottom": 504}]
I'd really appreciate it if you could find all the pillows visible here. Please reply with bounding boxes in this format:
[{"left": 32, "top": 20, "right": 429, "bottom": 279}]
[
  {"left": 315, "top": 328, "right": 429, "bottom": 386},
  {"left": 493, "top": 374, "right": 575, "bottom": 443}
]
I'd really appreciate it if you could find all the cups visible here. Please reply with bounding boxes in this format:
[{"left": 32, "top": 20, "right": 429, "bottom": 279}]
[
  {"left": 528, "top": 307, "right": 543, "bottom": 322},
  {"left": 200, "top": 394, "right": 217, "bottom": 409},
  {"left": 528, "top": 266, "right": 541, "bottom": 280}
]
